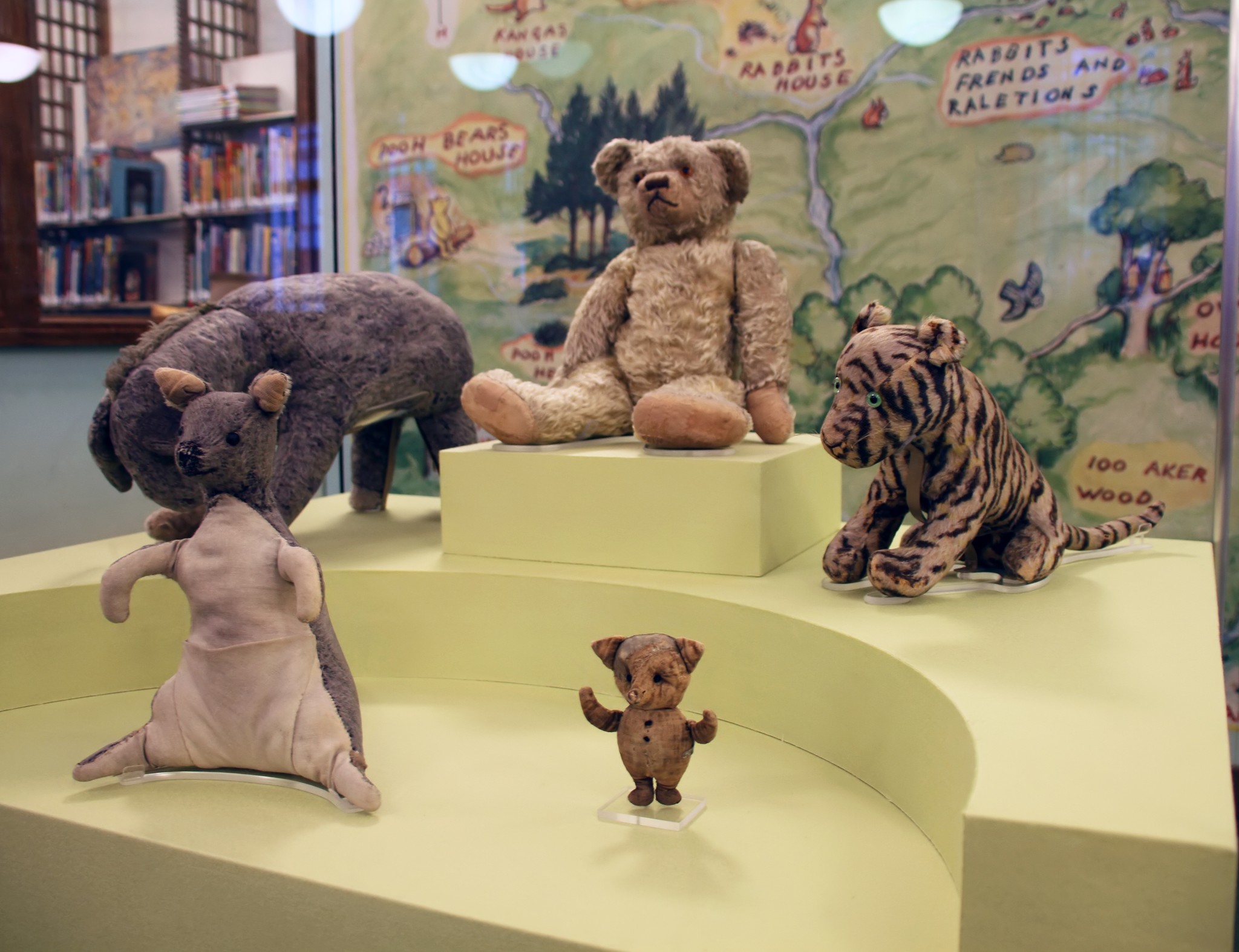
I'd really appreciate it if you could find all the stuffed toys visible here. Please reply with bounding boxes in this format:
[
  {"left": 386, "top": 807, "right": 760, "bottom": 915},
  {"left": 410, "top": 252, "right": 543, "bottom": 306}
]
[
  {"left": 458, "top": 135, "right": 795, "bottom": 449},
  {"left": 817, "top": 300, "right": 1166, "bottom": 599},
  {"left": 85, "top": 271, "right": 473, "bottom": 541},
  {"left": 72, "top": 366, "right": 381, "bottom": 816}
]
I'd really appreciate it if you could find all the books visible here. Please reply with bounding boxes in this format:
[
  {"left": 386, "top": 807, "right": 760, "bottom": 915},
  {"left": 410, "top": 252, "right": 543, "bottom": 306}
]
[{"left": 32, "top": 81, "right": 318, "bottom": 306}]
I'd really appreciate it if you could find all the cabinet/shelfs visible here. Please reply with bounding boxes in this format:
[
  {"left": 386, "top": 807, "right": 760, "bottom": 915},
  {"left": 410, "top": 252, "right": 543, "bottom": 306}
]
[{"left": 35, "top": 109, "right": 298, "bottom": 316}]
